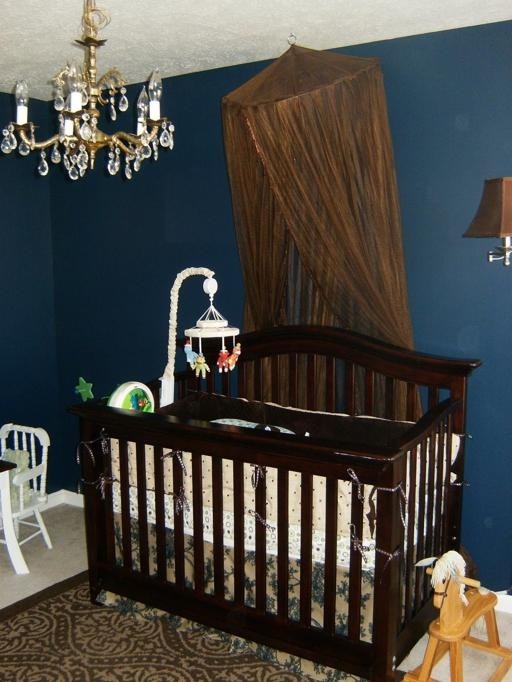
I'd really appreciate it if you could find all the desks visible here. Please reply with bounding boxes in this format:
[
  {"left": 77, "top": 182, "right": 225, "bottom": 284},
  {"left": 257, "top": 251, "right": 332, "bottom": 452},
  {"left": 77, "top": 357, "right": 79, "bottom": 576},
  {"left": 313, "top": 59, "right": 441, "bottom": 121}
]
[{"left": 0, "top": 460, "right": 31, "bottom": 577}]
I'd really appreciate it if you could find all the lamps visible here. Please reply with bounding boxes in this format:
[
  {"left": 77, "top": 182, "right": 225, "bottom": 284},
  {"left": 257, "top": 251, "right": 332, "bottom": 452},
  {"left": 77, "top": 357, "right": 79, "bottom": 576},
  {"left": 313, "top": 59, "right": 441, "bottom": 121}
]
[
  {"left": 460, "top": 176, "right": 512, "bottom": 268},
  {"left": 0, "top": 0, "right": 175, "bottom": 183}
]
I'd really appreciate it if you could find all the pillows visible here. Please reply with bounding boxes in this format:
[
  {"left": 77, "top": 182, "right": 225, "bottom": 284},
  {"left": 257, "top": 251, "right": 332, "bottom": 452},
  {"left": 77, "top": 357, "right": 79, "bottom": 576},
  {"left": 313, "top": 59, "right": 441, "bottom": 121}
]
[{"left": 1, "top": 448, "right": 31, "bottom": 510}]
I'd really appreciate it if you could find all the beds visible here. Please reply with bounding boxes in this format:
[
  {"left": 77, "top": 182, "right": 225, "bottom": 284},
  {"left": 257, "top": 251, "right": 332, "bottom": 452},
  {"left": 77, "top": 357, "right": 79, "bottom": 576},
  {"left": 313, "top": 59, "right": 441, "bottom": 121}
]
[{"left": 67, "top": 321, "right": 483, "bottom": 682}]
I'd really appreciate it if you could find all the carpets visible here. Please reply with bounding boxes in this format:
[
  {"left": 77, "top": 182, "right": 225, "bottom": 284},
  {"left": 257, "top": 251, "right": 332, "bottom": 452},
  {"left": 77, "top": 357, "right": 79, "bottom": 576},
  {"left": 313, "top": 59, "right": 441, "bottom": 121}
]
[{"left": 0, "top": 570, "right": 320, "bottom": 682}]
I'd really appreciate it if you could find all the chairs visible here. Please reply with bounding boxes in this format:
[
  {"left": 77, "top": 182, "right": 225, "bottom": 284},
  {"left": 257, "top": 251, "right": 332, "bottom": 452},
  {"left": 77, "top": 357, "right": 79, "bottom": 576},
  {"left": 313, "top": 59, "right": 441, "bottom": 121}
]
[
  {"left": 404, "top": 549, "right": 512, "bottom": 682},
  {"left": 0, "top": 422, "right": 53, "bottom": 551}
]
